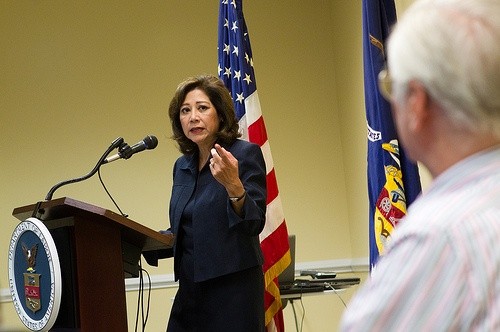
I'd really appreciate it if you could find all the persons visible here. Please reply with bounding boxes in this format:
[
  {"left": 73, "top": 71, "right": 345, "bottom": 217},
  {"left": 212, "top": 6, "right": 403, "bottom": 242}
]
[
  {"left": 341, "top": 0, "right": 500, "bottom": 332},
  {"left": 142, "top": 74, "right": 267, "bottom": 332}
]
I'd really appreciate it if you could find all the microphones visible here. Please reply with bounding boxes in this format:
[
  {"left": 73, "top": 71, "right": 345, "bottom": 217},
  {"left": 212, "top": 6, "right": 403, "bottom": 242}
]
[{"left": 103, "top": 136, "right": 158, "bottom": 164}]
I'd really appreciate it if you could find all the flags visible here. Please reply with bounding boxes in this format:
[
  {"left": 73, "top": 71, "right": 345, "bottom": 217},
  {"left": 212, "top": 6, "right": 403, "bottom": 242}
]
[
  {"left": 218, "top": 0, "right": 293, "bottom": 332},
  {"left": 361, "top": 0, "right": 423, "bottom": 272}
]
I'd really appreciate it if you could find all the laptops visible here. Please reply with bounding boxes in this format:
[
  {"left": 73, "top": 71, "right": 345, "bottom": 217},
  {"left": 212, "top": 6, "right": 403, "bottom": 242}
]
[
  {"left": 294, "top": 277, "right": 360, "bottom": 287},
  {"left": 276, "top": 235, "right": 295, "bottom": 289}
]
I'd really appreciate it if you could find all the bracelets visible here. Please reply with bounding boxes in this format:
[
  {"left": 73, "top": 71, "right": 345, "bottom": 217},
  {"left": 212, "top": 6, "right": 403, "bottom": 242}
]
[{"left": 229, "top": 188, "right": 247, "bottom": 202}]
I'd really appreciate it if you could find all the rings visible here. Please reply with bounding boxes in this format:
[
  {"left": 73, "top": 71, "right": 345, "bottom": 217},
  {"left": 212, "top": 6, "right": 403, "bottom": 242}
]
[{"left": 211, "top": 160, "right": 216, "bottom": 168}]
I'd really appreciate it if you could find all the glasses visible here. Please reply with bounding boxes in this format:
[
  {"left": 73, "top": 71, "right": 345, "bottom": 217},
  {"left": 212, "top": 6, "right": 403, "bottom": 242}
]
[{"left": 378, "top": 70, "right": 395, "bottom": 103}]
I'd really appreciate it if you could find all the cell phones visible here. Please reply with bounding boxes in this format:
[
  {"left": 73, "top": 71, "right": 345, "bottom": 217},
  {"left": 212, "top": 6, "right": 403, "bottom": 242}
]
[{"left": 299, "top": 271, "right": 336, "bottom": 278}]
